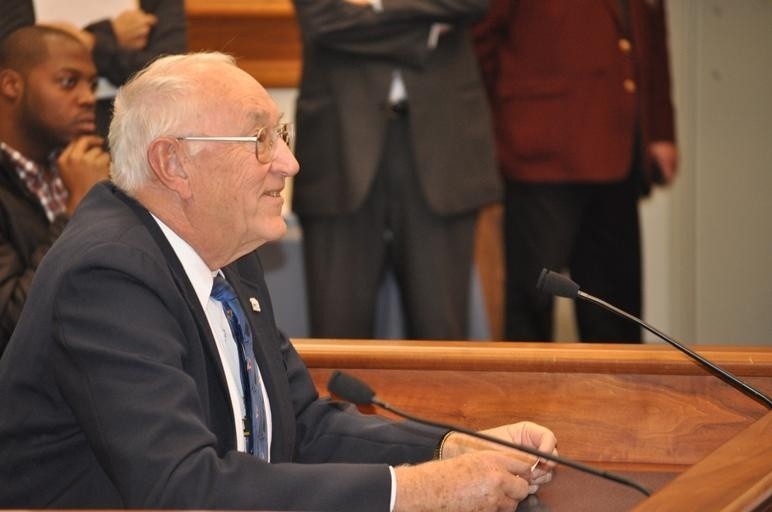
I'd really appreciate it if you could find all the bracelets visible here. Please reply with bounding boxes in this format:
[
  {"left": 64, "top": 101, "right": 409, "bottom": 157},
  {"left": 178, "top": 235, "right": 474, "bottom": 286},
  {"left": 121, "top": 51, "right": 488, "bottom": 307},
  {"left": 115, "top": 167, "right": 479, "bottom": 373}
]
[{"left": 432, "top": 430, "right": 454, "bottom": 460}]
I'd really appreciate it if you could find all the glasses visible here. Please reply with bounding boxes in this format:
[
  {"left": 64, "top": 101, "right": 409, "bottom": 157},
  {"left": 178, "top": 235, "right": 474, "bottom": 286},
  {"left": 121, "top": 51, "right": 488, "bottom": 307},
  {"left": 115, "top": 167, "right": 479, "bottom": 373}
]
[{"left": 175, "top": 122, "right": 295, "bottom": 166}]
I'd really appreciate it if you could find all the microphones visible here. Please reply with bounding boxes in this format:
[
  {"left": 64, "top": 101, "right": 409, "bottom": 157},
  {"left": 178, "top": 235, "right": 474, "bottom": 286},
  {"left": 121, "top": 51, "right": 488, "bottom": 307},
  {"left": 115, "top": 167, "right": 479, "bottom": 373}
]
[
  {"left": 532, "top": 267, "right": 771, "bottom": 411},
  {"left": 326, "top": 370, "right": 652, "bottom": 497}
]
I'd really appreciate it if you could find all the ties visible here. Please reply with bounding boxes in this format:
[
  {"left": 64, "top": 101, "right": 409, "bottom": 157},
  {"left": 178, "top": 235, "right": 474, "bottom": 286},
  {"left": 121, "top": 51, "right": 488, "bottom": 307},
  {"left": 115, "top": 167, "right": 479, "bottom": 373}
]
[{"left": 210, "top": 274, "right": 270, "bottom": 463}]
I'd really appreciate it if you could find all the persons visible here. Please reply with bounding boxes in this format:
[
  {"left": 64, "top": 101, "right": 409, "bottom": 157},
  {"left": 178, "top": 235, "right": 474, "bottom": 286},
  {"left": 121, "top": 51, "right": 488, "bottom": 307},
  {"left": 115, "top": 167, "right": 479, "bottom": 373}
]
[
  {"left": 289, "top": 1, "right": 505, "bottom": 340},
  {"left": 1, "top": 51, "right": 558, "bottom": 511},
  {"left": 469, "top": 1, "right": 678, "bottom": 345},
  {"left": 1, "top": 1, "right": 186, "bottom": 153},
  {"left": 0, "top": 26, "right": 111, "bottom": 355}
]
